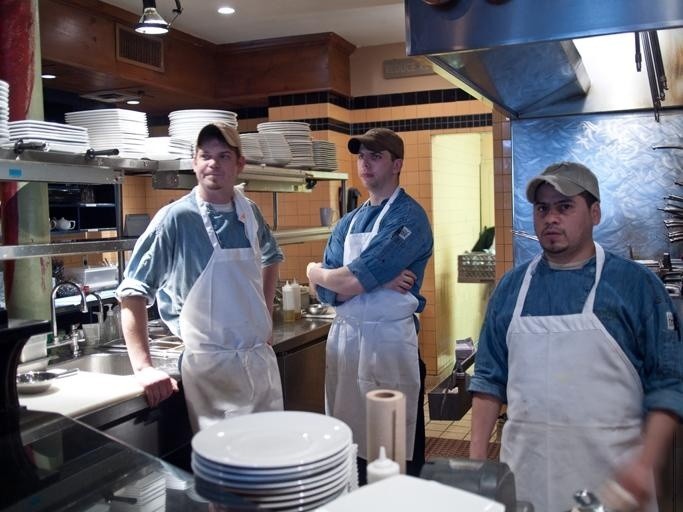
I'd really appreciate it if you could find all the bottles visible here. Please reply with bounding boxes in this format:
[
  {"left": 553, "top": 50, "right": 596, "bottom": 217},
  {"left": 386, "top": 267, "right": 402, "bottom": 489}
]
[
  {"left": 282, "top": 277, "right": 301, "bottom": 310},
  {"left": 366, "top": 446, "right": 400, "bottom": 484}
]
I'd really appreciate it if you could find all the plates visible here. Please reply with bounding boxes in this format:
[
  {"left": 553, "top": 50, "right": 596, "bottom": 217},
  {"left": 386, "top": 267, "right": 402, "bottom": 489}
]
[
  {"left": 8, "top": 119, "right": 90, "bottom": 155},
  {"left": 110, "top": 469, "right": 186, "bottom": 512},
  {"left": 190, "top": 411, "right": 354, "bottom": 512},
  {"left": 65, "top": 109, "right": 149, "bottom": 161},
  {"left": 241, "top": 131, "right": 293, "bottom": 167},
  {"left": 169, "top": 108, "right": 238, "bottom": 159},
  {"left": 311, "top": 140, "right": 339, "bottom": 171},
  {"left": 257, "top": 122, "right": 316, "bottom": 170},
  {"left": 0, "top": 81, "right": 10, "bottom": 146},
  {"left": 148, "top": 137, "right": 193, "bottom": 160},
  {"left": 238, "top": 136, "right": 264, "bottom": 162}
]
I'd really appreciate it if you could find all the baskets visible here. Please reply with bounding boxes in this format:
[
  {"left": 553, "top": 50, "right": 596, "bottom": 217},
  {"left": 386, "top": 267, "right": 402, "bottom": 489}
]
[{"left": 457, "top": 251, "right": 495, "bottom": 284}]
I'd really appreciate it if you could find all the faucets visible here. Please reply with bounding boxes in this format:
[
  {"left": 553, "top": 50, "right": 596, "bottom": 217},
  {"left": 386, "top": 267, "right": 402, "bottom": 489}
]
[
  {"left": 48, "top": 279, "right": 86, "bottom": 340},
  {"left": 79, "top": 290, "right": 105, "bottom": 313}
]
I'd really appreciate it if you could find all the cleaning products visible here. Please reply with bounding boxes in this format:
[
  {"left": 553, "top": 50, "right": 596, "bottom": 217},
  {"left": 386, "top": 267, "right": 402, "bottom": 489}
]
[
  {"left": 100, "top": 303, "right": 120, "bottom": 340},
  {"left": 92, "top": 310, "right": 106, "bottom": 338}
]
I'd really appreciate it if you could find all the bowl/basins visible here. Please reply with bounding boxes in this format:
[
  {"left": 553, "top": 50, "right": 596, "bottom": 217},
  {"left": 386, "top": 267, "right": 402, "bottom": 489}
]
[{"left": 306, "top": 304, "right": 329, "bottom": 314}]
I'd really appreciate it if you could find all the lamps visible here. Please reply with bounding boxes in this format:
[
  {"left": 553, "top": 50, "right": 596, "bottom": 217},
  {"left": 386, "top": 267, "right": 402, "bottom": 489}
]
[{"left": 135, "top": 0, "right": 182, "bottom": 37}]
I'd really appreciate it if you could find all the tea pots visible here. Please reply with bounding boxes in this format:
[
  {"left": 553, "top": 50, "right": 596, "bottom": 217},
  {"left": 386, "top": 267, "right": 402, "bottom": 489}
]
[{"left": 49, "top": 217, "right": 76, "bottom": 231}]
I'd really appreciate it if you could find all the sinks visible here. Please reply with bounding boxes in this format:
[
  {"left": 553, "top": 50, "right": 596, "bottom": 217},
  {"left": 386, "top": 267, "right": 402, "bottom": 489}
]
[{"left": 50, "top": 350, "right": 177, "bottom": 380}]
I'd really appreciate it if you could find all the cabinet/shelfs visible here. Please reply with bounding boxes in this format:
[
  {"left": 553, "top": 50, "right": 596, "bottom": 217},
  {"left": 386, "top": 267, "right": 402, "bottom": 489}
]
[{"left": 0, "top": 147, "right": 348, "bottom": 446}]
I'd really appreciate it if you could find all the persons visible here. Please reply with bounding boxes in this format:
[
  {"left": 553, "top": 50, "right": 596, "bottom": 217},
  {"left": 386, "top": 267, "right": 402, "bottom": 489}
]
[
  {"left": 113, "top": 121, "right": 286, "bottom": 439},
  {"left": 306, "top": 126, "right": 435, "bottom": 487},
  {"left": 468, "top": 160, "right": 683, "bottom": 511}
]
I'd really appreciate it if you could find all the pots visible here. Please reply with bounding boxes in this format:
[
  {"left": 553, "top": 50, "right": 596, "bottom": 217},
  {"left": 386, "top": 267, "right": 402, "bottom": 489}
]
[{"left": 15, "top": 367, "right": 80, "bottom": 393}]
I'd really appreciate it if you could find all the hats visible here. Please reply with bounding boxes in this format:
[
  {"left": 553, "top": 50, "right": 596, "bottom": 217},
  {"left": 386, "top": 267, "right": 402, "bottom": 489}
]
[
  {"left": 527, "top": 161, "right": 600, "bottom": 205},
  {"left": 348, "top": 128, "right": 404, "bottom": 159},
  {"left": 197, "top": 121, "right": 241, "bottom": 158}
]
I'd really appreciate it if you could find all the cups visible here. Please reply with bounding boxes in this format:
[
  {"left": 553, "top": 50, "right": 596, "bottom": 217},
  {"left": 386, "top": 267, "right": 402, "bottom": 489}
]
[{"left": 320, "top": 207, "right": 338, "bottom": 226}]
